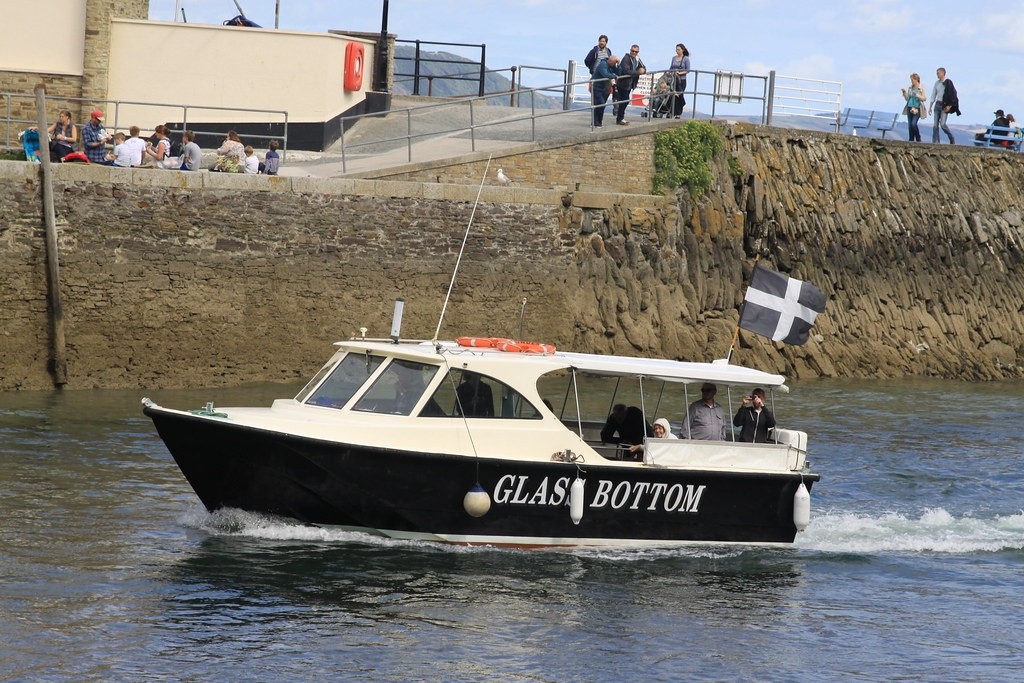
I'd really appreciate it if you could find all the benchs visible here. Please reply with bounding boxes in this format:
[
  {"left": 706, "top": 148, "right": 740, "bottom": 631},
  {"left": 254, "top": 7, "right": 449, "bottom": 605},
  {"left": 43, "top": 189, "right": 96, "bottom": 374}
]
[
  {"left": 830, "top": 108, "right": 899, "bottom": 134},
  {"left": 970, "top": 126, "right": 1024, "bottom": 148}
]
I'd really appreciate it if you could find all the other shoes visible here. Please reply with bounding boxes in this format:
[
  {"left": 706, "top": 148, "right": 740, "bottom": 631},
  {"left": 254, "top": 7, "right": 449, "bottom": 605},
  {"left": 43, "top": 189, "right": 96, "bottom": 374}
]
[
  {"left": 666, "top": 114, "right": 672, "bottom": 118},
  {"left": 652, "top": 109, "right": 656, "bottom": 112},
  {"left": 617, "top": 119, "right": 629, "bottom": 125},
  {"left": 645, "top": 107, "right": 649, "bottom": 110},
  {"left": 675, "top": 115, "right": 680, "bottom": 119}
]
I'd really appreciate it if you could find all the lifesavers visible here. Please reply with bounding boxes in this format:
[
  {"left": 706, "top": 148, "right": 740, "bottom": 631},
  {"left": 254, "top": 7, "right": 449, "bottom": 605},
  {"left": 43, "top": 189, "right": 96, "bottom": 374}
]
[
  {"left": 457, "top": 334, "right": 513, "bottom": 347},
  {"left": 497, "top": 340, "right": 557, "bottom": 354}
]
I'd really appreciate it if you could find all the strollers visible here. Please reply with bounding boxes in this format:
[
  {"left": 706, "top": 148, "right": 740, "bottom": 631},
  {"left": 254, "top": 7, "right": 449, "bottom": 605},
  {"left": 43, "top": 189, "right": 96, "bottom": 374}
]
[{"left": 641, "top": 69, "right": 682, "bottom": 119}]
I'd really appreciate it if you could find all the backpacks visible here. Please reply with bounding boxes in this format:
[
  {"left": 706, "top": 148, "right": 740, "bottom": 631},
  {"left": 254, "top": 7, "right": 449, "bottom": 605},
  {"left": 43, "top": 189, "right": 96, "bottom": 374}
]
[{"left": 161, "top": 138, "right": 184, "bottom": 156}]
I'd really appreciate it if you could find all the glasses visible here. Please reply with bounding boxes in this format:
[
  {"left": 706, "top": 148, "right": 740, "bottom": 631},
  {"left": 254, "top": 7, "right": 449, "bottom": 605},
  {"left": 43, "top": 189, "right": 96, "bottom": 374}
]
[
  {"left": 701, "top": 387, "right": 713, "bottom": 393},
  {"left": 631, "top": 49, "right": 639, "bottom": 53}
]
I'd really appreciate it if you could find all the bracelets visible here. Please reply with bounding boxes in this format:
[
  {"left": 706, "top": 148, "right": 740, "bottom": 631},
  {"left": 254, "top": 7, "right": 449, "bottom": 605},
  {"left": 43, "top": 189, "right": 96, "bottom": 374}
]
[{"left": 65, "top": 136, "right": 66, "bottom": 141}]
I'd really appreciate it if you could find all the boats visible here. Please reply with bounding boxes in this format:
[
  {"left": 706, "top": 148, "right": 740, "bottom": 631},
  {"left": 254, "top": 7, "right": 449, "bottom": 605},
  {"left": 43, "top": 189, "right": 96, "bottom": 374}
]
[{"left": 142, "top": 152, "right": 821, "bottom": 552}]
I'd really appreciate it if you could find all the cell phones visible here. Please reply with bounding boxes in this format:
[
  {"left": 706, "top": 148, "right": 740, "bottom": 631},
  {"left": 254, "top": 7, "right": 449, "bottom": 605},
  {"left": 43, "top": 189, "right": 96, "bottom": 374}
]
[{"left": 749, "top": 396, "right": 756, "bottom": 400}]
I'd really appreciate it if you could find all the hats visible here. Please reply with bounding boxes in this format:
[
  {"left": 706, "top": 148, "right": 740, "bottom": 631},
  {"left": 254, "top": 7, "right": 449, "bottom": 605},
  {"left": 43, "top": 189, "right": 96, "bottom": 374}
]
[
  {"left": 994, "top": 110, "right": 1004, "bottom": 116},
  {"left": 92, "top": 110, "right": 105, "bottom": 121}
]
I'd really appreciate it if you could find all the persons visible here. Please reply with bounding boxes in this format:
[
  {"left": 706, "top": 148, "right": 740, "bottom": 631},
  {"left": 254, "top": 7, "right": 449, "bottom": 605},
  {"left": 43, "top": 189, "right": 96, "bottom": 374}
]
[
  {"left": 534, "top": 399, "right": 553, "bottom": 417},
  {"left": 733, "top": 388, "right": 776, "bottom": 443},
  {"left": 928, "top": 68, "right": 955, "bottom": 144},
  {"left": 666, "top": 44, "right": 690, "bottom": 119},
  {"left": 395, "top": 368, "right": 424, "bottom": 416},
  {"left": 28, "top": 111, "right": 76, "bottom": 161},
  {"left": 209, "top": 131, "right": 244, "bottom": 172},
  {"left": 258, "top": 141, "right": 279, "bottom": 175},
  {"left": 585, "top": 35, "right": 612, "bottom": 74},
  {"left": 244, "top": 145, "right": 259, "bottom": 174},
  {"left": 601, "top": 404, "right": 654, "bottom": 462},
  {"left": 456, "top": 374, "right": 494, "bottom": 416},
  {"left": 82, "top": 110, "right": 202, "bottom": 170},
  {"left": 901, "top": 74, "right": 927, "bottom": 142},
  {"left": 629, "top": 418, "right": 678, "bottom": 452},
  {"left": 616, "top": 45, "right": 646, "bottom": 125},
  {"left": 590, "top": 55, "right": 621, "bottom": 128},
  {"left": 974, "top": 111, "right": 1017, "bottom": 147},
  {"left": 680, "top": 383, "right": 726, "bottom": 440},
  {"left": 653, "top": 80, "right": 670, "bottom": 111}
]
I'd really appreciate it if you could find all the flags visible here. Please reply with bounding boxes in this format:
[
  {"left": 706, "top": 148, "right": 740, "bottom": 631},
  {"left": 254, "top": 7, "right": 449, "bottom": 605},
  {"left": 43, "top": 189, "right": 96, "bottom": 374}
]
[{"left": 740, "top": 265, "right": 827, "bottom": 345}]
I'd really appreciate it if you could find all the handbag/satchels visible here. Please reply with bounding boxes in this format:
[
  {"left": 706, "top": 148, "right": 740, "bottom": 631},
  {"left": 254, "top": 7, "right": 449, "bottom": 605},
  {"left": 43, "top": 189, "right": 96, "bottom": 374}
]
[
  {"left": 910, "top": 106, "right": 919, "bottom": 114},
  {"left": 215, "top": 155, "right": 240, "bottom": 173}
]
